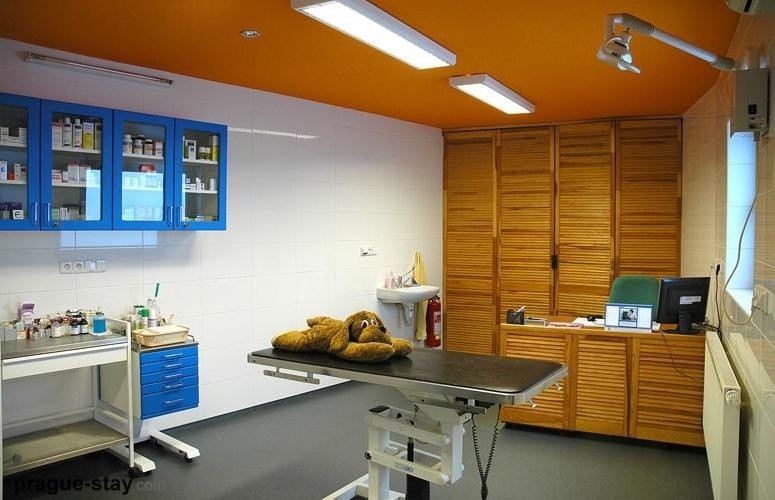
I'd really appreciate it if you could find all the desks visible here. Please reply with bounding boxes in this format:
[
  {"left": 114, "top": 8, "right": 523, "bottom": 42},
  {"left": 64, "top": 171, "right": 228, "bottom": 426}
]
[{"left": 498, "top": 317, "right": 705, "bottom": 446}]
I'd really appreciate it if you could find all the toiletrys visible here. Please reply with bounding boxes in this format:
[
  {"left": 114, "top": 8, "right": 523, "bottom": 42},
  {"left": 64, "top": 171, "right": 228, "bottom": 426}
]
[{"left": 386, "top": 271, "right": 396, "bottom": 288}]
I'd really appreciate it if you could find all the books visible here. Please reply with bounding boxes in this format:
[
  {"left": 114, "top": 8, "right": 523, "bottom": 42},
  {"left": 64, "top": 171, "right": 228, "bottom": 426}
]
[{"left": 545, "top": 321, "right": 584, "bottom": 330}]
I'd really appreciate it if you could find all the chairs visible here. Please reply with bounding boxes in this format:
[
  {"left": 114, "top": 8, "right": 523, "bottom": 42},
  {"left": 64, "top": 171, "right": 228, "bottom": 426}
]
[{"left": 607, "top": 273, "right": 658, "bottom": 323}]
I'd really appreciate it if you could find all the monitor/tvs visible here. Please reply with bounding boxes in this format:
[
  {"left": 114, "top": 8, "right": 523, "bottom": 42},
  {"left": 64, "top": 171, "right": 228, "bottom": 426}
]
[{"left": 656, "top": 277, "right": 710, "bottom": 335}]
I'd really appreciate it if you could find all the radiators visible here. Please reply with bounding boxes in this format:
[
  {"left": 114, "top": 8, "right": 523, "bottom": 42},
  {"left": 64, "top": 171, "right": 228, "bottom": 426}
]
[{"left": 701, "top": 331, "right": 742, "bottom": 500}]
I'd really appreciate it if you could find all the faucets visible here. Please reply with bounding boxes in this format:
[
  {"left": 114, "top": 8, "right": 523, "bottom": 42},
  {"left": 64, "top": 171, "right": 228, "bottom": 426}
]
[{"left": 397, "top": 266, "right": 415, "bottom": 287}]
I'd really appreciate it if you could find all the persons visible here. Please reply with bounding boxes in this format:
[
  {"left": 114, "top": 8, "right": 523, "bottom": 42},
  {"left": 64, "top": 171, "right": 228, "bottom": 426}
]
[
  {"left": 627, "top": 309, "right": 635, "bottom": 319},
  {"left": 271, "top": 311, "right": 416, "bottom": 364}
]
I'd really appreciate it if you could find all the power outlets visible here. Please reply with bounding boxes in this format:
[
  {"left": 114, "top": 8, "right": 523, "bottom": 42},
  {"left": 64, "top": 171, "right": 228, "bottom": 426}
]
[
  {"left": 59, "top": 261, "right": 74, "bottom": 273},
  {"left": 74, "top": 262, "right": 85, "bottom": 273}
]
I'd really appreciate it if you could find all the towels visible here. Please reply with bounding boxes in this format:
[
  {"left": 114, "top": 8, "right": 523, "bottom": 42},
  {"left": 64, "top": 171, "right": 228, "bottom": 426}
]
[{"left": 413, "top": 252, "right": 429, "bottom": 341}]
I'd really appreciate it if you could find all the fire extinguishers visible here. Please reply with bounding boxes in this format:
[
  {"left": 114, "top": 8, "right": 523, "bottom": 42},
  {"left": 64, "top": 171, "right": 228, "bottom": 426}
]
[{"left": 427, "top": 295, "right": 441, "bottom": 347}]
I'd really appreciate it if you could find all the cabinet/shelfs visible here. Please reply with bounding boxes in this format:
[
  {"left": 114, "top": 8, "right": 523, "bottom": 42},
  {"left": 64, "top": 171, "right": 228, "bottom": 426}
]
[
  {"left": 0, "top": 92, "right": 113, "bottom": 231},
  {"left": 112, "top": 108, "right": 227, "bottom": 230},
  {"left": 94, "top": 336, "right": 201, "bottom": 473}
]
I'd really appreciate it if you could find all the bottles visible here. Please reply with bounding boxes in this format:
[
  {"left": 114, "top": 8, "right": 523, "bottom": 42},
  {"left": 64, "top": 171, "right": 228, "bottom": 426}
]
[
  {"left": 122, "top": 135, "right": 153, "bottom": 155},
  {"left": 142, "top": 308, "right": 156, "bottom": 328},
  {"left": 26, "top": 312, "right": 106, "bottom": 340},
  {"left": 62, "top": 117, "right": 82, "bottom": 149}
]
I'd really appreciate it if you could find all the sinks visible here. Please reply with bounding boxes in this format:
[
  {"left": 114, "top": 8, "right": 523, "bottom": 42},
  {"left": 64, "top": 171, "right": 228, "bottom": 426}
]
[{"left": 377, "top": 284, "right": 440, "bottom": 303}]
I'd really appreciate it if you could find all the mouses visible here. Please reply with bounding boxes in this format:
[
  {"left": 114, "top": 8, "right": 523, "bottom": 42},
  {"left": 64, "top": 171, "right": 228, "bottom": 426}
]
[{"left": 587, "top": 315, "right": 595, "bottom": 321}]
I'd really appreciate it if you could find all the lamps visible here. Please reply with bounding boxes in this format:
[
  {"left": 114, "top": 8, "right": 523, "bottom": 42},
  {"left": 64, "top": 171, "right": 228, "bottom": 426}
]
[
  {"left": 287, "top": 0, "right": 456, "bottom": 70},
  {"left": 594, "top": 10, "right": 747, "bottom": 77},
  {"left": 24, "top": 51, "right": 173, "bottom": 89},
  {"left": 447, "top": 74, "right": 536, "bottom": 115}
]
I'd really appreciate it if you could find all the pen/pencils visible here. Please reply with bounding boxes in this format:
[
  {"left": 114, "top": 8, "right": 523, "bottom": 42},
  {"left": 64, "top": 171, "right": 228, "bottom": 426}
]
[{"left": 511, "top": 306, "right": 526, "bottom": 322}]
